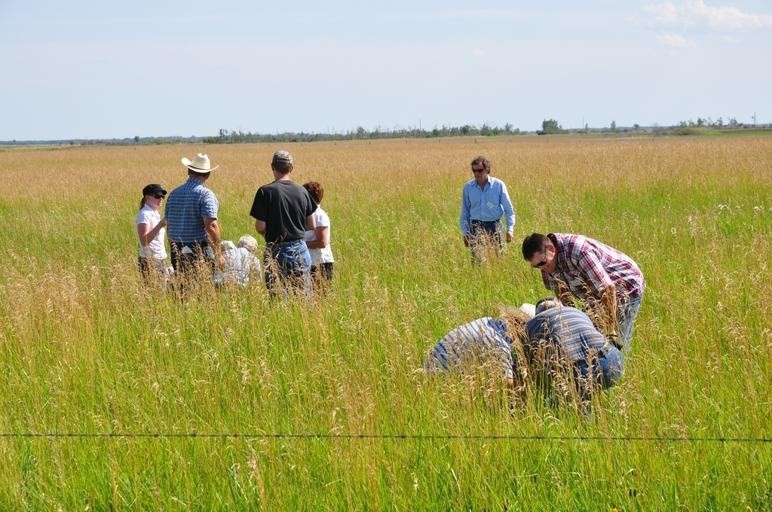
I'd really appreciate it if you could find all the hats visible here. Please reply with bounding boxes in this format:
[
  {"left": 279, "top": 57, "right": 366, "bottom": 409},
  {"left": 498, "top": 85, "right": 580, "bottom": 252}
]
[
  {"left": 273, "top": 150, "right": 293, "bottom": 164},
  {"left": 518, "top": 303, "right": 536, "bottom": 319},
  {"left": 181, "top": 153, "right": 219, "bottom": 173},
  {"left": 143, "top": 184, "right": 167, "bottom": 196}
]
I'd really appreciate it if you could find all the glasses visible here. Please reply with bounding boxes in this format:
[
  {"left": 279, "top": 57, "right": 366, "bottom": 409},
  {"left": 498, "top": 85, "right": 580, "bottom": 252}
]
[
  {"left": 154, "top": 194, "right": 165, "bottom": 199},
  {"left": 532, "top": 247, "right": 547, "bottom": 268},
  {"left": 473, "top": 169, "right": 483, "bottom": 173}
]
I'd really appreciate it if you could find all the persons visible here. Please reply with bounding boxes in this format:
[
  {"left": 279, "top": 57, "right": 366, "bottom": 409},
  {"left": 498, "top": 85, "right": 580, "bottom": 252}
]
[
  {"left": 426, "top": 303, "right": 536, "bottom": 420},
  {"left": 522, "top": 298, "right": 626, "bottom": 406},
  {"left": 136, "top": 150, "right": 337, "bottom": 302},
  {"left": 459, "top": 156, "right": 515, "bottom": 267},
  {"left": 522, "top": 232, "right": 646, "bottom": 354}
]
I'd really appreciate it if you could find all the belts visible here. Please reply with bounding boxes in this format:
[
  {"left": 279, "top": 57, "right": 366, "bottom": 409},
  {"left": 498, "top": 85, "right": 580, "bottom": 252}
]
[
  {"left": 571, "top": 343, "right": 612, "bottom": 367},
  {"left": 472, "top": 219, "right": 499, "bottom": 224}
]
